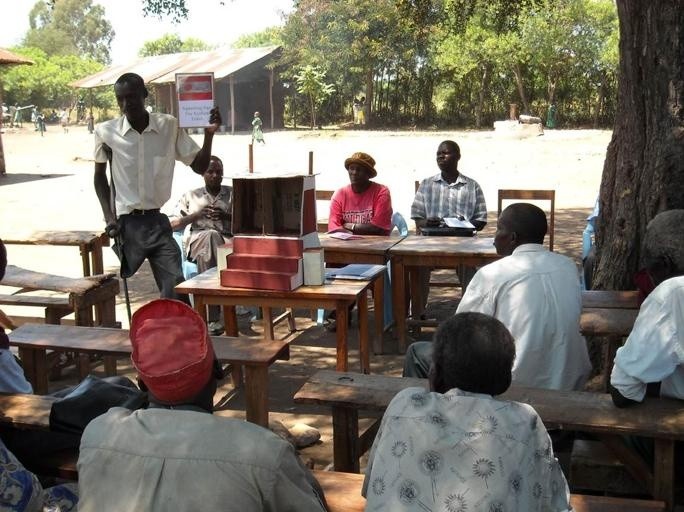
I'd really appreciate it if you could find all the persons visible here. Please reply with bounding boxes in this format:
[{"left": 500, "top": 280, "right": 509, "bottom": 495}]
[
  {"left": 76, "top": 297, "right": 333, "bottom": 512},
  {"left": 410, "top": 140, "right": 488, "bottom": 320},
  {"left": 352, "top": 99, "right": 362, "bottom": 129},
  {"left": 544, "top": 100, "right": 557, "bottom": 128},
  {"left": 0, "top": 101, "right": 95, "bottom": 137},
  {"left": 606, "top": 210, "right": 684, "bottom": 464},
  {"left": 251, "top": 111, "right": 266, "bottom": 146},
  {"left": 0, "top": 237, "right": 145, "bottom": 470},
  {"left": 581, "top": 196, "right": 599, "bottom": 290},
  {"left": 92, "top": 71, "right": 224, "bottom": 309},
  {"left": 357, "top": 96, "right": 366, "bottom": 129},
  {"left": 323, "top": 151, "right": 398, "bottom": 338},
  {"left": 363, "top": 312, "right": 571, "bottom": 512},
  {"left": 401, "top": 202, "right": 592, "bottom": 395},
  {"left": 168, "top": 156, "right": 235, "bottom": 336}
]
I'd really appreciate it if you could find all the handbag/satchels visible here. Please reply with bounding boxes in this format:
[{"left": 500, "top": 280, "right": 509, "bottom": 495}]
[{"left": 49, "top": 373, "right": 147, "bottom": 435}]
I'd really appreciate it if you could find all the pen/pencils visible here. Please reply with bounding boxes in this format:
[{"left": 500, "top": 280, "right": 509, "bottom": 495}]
[{"left": 330, "top": 274, "right": 361, "bottom": 276}]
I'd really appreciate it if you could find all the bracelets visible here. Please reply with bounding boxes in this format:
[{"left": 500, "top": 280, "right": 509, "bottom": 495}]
[{"left": 351, "top": 222, "right": 355, "bottom": 232}]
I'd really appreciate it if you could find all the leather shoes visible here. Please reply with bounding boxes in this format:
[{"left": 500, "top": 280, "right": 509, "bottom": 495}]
[
  {"left": 236, "top": 308, "right": 252, "bottom": 319},
  {"left": 207, "top": 321, "right": 224, "bottom": 335}
]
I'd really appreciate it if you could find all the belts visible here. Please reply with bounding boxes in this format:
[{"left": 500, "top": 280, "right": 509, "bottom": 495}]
[{"left": 129, "top": 209, "right": 160, "bottom": 216}]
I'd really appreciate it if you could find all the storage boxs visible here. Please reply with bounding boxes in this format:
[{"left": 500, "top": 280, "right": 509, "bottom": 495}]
[{"left": 230, "top": 175, "right": 318, "bottom": 237}]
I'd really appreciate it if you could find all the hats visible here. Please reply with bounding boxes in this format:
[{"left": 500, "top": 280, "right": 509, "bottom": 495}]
[
  {"left": 345, "top": 152, "right": 377, "bottom": 178},
  {"left": 129, "top": 299, "right": 214, "bottom": 401}
]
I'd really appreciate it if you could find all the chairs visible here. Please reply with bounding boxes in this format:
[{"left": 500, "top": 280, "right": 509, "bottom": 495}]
[{"left": 315, "top": 180, "right": 556, "bottom": 288}]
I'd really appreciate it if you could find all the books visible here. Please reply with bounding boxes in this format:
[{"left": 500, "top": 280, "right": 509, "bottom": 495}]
[{"left": 323, "top": 262, "right": 387, "bottom": 281}]
